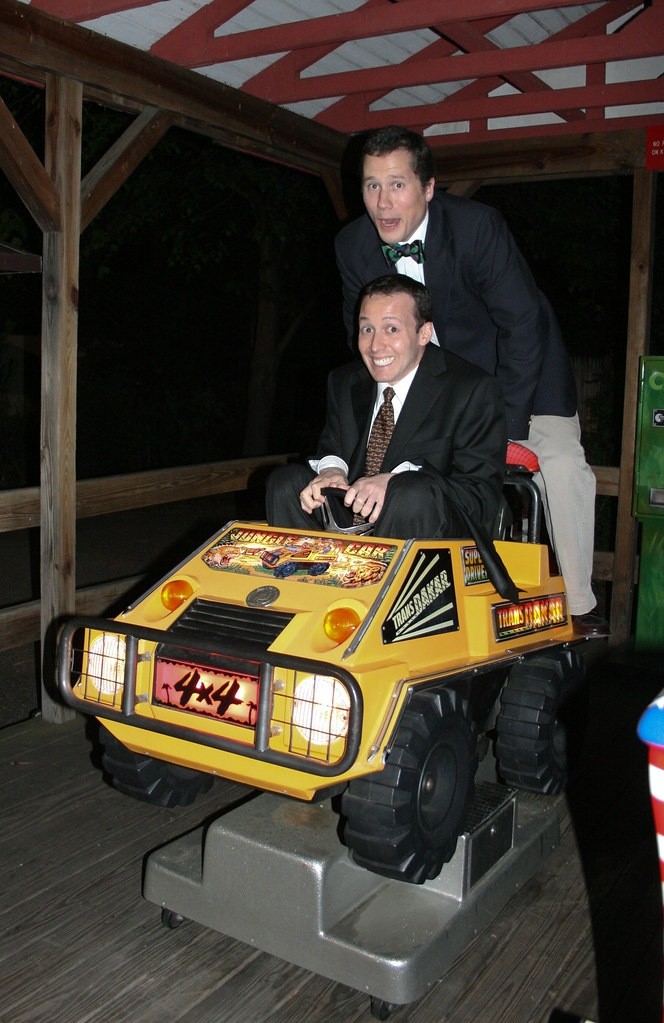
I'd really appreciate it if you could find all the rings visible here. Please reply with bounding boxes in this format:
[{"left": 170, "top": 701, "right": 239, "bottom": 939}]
[{"left": 355, "top": 499, "right": 364, "bottom": 504}]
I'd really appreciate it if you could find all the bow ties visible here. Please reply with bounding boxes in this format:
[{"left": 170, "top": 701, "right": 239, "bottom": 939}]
[{"left": 382, "top": 239, "right": 425, "bottom": 270}]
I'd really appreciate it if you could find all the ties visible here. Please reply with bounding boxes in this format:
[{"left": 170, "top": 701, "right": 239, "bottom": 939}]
[{"left": 352, "top": 385, "right": 395, "bottom": 527}]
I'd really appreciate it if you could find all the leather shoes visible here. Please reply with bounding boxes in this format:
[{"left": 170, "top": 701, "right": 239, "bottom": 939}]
[{"left": 573, "top": 606, "right": 610, "bottom": 636}]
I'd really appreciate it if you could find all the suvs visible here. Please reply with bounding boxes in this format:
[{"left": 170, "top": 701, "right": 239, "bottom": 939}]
[{"left": 41, "top": 439, "right": 611, "bottom": 886}]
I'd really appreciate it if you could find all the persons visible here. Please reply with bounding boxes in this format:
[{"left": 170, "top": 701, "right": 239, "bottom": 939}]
[
  {"left": 264, "top": 274, "right": 509, "bottom": 540},
  {"left": 335, "top": 125, "right": 603, "bottom": 633}
]
